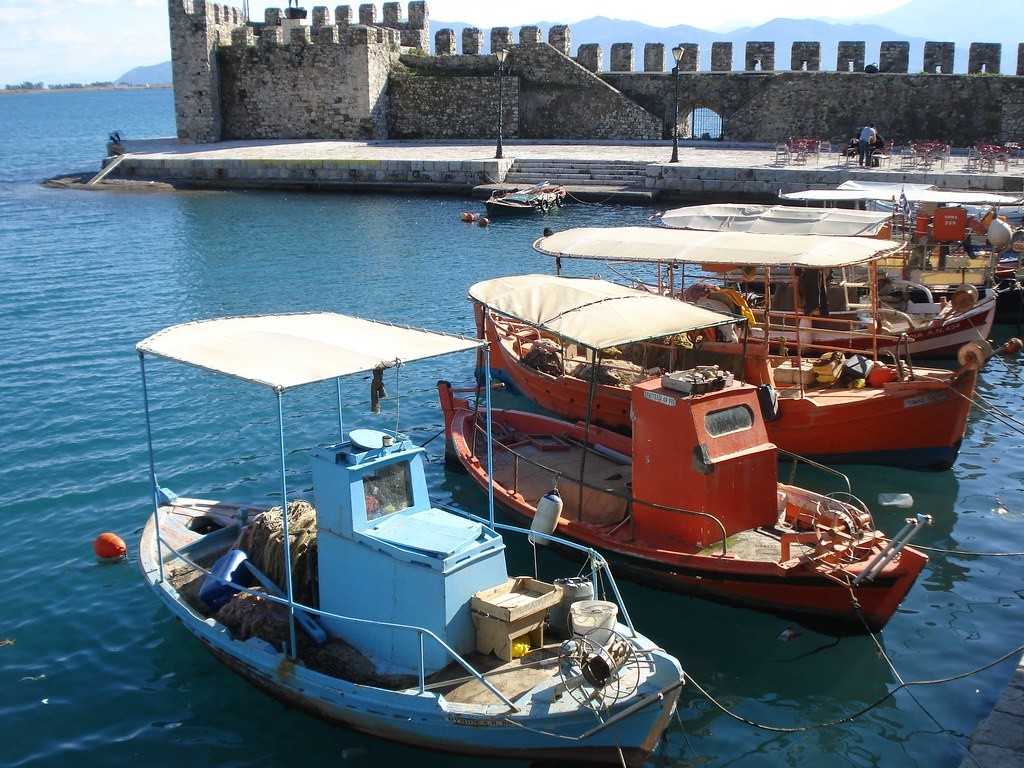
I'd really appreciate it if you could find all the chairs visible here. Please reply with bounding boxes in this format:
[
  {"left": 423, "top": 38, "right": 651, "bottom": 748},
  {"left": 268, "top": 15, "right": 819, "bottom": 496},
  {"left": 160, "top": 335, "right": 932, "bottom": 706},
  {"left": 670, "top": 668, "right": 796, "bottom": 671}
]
[{"left": 772, "top": 136, "right": 1024, "bottom": 174}]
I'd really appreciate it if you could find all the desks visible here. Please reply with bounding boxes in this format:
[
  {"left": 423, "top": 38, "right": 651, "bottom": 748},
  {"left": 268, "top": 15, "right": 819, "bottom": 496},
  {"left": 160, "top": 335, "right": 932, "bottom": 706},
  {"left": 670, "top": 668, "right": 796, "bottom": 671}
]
[{"left": 792, "top": 144, "right": 808, "bottom": 166}]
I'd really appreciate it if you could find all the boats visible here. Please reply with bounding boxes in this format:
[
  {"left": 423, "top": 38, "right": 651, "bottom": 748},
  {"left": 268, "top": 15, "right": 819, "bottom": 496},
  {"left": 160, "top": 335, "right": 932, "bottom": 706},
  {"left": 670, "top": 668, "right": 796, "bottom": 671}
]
[
  {"left": 436, "top": 272, "right": 932, "bottom": 627},
  {"left": 131, "top": 309, "right": 688, "bottom": 768},
  {"left": 641, "top": 177, "right": 1024, "bottom": 360},
  {"left": 531, "top": 226, "right": 1022, "bottom": 463},
  {"left": 481, "top": 184, "right": 570, "bottom": 217}
]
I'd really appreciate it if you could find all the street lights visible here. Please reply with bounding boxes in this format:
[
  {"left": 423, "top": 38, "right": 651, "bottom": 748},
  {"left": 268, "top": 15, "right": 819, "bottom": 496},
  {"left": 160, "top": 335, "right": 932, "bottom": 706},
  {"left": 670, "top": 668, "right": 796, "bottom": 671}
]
[
  {"left": 668, "top": 46, "right": 687, "bottom": 162},
  {"left": 493, "top": 48, "right": 511, "bottom": 159}
]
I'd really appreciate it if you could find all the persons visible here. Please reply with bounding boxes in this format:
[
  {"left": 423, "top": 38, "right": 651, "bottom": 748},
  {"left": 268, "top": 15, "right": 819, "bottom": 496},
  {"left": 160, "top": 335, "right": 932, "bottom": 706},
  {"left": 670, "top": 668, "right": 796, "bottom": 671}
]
[{"left": 858, "top": 122, "right": 883, "bottom": 169}]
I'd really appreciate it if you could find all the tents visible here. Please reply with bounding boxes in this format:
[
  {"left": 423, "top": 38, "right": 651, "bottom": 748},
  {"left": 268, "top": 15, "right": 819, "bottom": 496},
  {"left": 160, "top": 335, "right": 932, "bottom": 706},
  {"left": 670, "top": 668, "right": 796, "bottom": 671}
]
[{"left": 133, "top": 185, "right": 1021, "bottom": 663}]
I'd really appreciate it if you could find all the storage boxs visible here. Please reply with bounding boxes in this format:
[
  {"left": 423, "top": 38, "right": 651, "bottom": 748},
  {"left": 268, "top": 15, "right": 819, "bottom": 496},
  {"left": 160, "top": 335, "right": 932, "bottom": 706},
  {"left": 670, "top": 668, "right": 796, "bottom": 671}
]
[
  {"left": 199, "top": 549, "right": 251, "bottom": 612},
  {"left": 841, "top": 354, "right": 875, "bottom": 386},
  {"left": 812, "top": 351, "right": 846, "bottom": 385},
  {"left": 660, "top": 368, "right": 734, "bottom": 394},
  {"left": 774, "top": 358, "right": 816, "bottom": 384},
  {"left": 469, "top": 575, "right": 565, "bottom": 623}
]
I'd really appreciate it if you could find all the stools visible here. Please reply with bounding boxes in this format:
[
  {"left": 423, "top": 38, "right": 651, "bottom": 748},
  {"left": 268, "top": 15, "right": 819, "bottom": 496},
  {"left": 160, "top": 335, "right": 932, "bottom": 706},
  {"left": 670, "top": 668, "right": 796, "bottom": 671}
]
[{"left": 471, "top": 608, "right": 547, "bottom": 661}]
